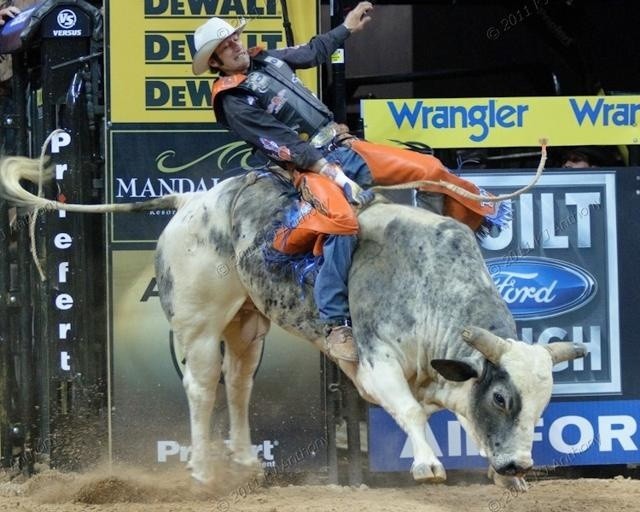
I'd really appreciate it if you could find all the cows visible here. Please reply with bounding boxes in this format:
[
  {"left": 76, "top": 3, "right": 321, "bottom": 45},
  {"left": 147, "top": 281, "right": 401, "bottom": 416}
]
[{"left": 1, "top": 155, "right": 589, "bottom": 493}]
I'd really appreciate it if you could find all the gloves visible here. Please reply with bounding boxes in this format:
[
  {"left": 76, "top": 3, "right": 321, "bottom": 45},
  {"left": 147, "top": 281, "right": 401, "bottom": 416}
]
[
  {"left": 342, "top": 1, "right": 376, "bottom": 35},
  {"left": 321, "top": 161, "right": 374, "bottom": 206}
]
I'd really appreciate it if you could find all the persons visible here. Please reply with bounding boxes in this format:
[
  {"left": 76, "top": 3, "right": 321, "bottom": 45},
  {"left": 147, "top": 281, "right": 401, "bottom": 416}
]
[
  {"left": 190, "top": 0, "right": 505, "bottom": 363},
  {"left": 0, "top": 0, "right": 23, "bottom": 83},
  {"left": 549, "top": 145, "right": 603, "bottom": 170}
]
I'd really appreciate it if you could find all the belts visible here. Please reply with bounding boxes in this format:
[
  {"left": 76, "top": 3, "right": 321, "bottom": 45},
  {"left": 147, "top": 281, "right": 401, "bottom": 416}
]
[{"left": 310, "top": 122, "right": 348, "bottom": 151}]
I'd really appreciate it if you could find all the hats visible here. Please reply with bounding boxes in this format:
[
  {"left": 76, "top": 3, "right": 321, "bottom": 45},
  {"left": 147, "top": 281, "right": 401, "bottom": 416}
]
[{"left": 191, "top": 15, "right": 248, "bottom": 76}]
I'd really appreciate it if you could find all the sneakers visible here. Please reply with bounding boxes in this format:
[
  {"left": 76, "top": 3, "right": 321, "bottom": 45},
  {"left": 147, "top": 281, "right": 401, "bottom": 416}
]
[{"left": 326, "top": 326, "right": 360, "bottom": 365}]
